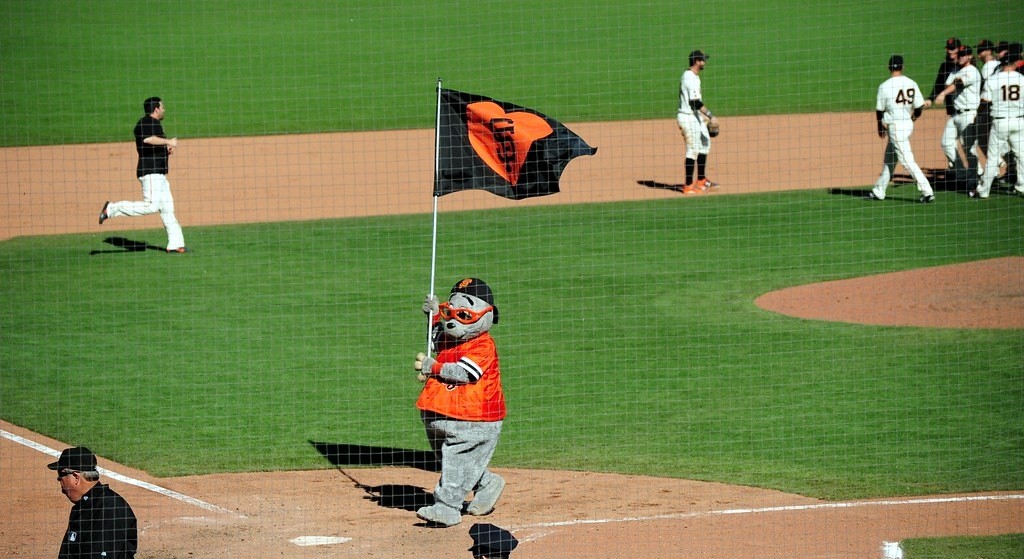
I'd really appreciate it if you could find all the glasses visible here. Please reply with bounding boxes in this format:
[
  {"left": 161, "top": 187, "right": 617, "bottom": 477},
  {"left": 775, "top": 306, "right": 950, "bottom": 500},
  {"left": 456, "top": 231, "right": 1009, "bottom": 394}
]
[{"left": 57, "top": 470, "right": 73, "bottom": 477}]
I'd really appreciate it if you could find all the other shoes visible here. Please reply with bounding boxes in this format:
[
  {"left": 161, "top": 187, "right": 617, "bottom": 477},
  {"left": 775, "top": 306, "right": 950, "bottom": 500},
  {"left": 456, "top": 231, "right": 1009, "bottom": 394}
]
[
  {"left": 998, "top": 162, "right": 1006, "bottom": 178},
  {"left": 919, "top": 194, "right": 935, "bottom": 203},
  {"left": 167, "top": 247, "right": 187, "bottom": 253},
  {"left": 969, "top": 191, "right": 988, "bottom": 199},
  {"left": 1006, "top": 189, "right": 1023, "bottom": 195},
  {"left": 98, "top": 200, "right": 109, "bottom": 225},
  {"left": 870, "top": 192, "right": 884, "bottom": 200}
]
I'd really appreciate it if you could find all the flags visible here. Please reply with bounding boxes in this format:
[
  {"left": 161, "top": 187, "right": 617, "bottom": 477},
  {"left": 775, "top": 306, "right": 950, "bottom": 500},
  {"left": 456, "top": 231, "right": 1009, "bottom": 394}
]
[{"left": 435, "top": 88, "right": 597, "bottom": 200}]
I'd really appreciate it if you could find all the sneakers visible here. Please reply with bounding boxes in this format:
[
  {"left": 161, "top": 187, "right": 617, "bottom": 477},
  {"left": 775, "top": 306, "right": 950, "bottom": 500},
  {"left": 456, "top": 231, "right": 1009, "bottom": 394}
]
[
  {"left": 696, "top": 179, "right": 720, "bottom": 190},
  {"left": 683, "top": 183, "right": 705, "bottom": 194}
]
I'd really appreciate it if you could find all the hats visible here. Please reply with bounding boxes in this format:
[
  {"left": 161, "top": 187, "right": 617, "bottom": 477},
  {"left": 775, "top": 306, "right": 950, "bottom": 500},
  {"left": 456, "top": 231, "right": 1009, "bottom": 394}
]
[
  {"left": 689, "top": 50, "right": 710, "bottom": 62},
  {"left": 888, "top": 55, "right": 903, "bottom": 67},
  {"left": 946, "top": 36, "right": 1024, "bottom": 66},
  {"left": 47, "top": 447, "right": 97, "bottom": 471},
  {"left": 468, "top": 523, "right": 518, "bottom": 554}
]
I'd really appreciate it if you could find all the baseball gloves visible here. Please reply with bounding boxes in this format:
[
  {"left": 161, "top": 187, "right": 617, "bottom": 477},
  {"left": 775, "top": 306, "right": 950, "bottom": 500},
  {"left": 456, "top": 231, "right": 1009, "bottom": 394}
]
[{"left": 707, "top": 122, "right": 720, "bottom": 137}]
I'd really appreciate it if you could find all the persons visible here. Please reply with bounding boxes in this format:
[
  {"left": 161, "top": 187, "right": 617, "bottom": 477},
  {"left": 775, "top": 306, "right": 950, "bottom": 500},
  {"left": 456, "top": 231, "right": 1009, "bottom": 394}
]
[
  {"left": 676, "top": 50, "right": 720, "bottom": 194},
  {"left": 923, "top": 37, "right": 1024, "bottom": 200},
  {"left": 99, "top": 97, "right": 193, "bottom": 252},
  {"left": 47, "top": 446, "right": 137, "bottom": 559},
  {"left": 865, "top": 55, "right": 935, "bottom": 204},
  {"left": 467, "top": 523, "right": 518, "bottom": 559}
]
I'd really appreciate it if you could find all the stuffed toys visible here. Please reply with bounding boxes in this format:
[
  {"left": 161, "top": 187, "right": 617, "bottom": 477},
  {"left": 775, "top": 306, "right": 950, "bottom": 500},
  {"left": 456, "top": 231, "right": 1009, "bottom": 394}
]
[{"left": 414, "top": 277, "right": 507, "bottom": 524}]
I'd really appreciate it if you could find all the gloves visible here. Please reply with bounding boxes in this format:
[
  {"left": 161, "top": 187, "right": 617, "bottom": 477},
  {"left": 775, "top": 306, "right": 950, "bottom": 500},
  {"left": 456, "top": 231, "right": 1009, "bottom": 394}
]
[{"left": 878, "top": 121, "right": 887, "bottom": 137}]
